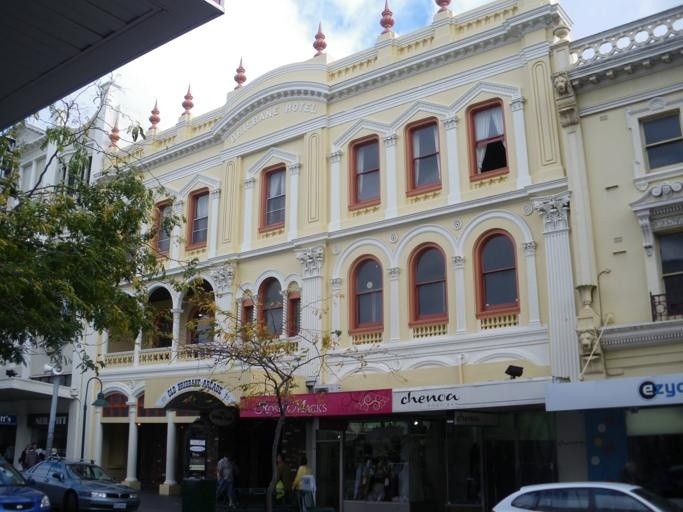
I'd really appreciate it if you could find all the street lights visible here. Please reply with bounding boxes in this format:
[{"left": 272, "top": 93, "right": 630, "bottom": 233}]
[{"left": 81, "top": 376, "right": 109, "bottom": 460}]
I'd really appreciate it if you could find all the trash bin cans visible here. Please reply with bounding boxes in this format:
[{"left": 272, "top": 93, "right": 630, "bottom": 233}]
[{"left": 180, "top": 479, "right": 217, "bottom": 512}]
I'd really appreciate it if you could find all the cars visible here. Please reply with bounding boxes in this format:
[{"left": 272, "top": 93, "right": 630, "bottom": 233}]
[
  {"left": 492, "top": 481, "right": 682, "bottom": 512},
  {"left": 0, "top": 457, "right": 140, "bottom": 512}
]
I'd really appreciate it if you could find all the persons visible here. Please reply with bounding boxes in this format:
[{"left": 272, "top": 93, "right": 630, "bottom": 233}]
[
  {"left": 214, "top": 450, "right": 236, "bottom": 508},
  {"left": 3, "top": 441, "right": 58, "bottom": 472},
  {"left": 276, "top": 452, "right": 290, "bottom": 508},
  {"left": 290, "top": 456, "right": 315, "bottom": 511}
]
